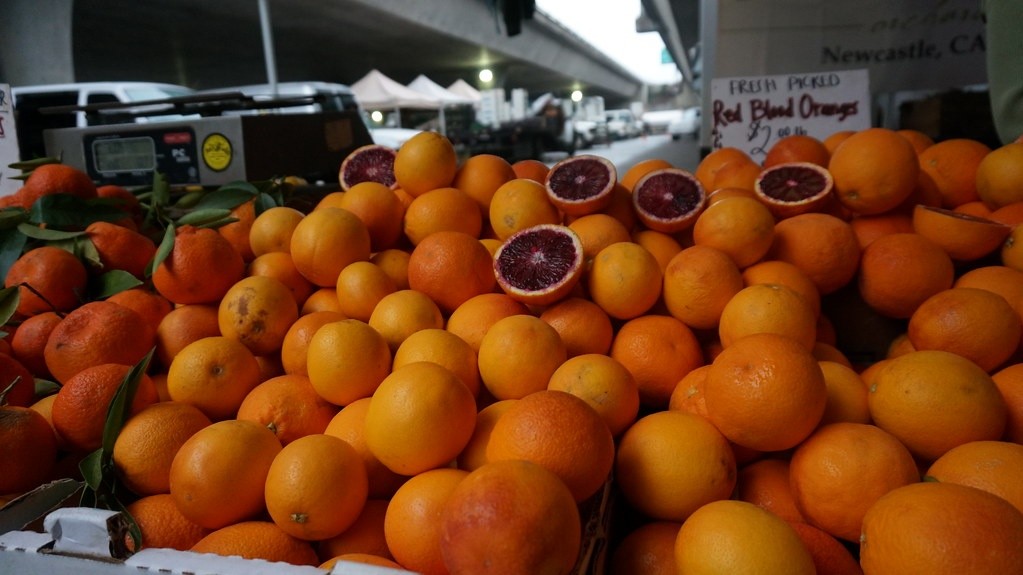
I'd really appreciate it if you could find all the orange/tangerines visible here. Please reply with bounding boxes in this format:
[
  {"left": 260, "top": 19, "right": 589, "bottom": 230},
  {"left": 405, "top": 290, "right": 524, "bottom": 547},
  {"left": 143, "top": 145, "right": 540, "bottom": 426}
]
[
  {"left": 0, "top": 151, "right": 275, "bottom": 495},
  {"left": 112, "top": 131, "right": 1023, "bottom": 574}
]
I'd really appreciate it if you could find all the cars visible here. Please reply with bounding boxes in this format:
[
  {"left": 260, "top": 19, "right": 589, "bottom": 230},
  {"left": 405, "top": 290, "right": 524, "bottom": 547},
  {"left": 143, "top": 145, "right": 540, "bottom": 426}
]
[{"left": 9, "top": 75, "right": 707, "bottom": 182}]
